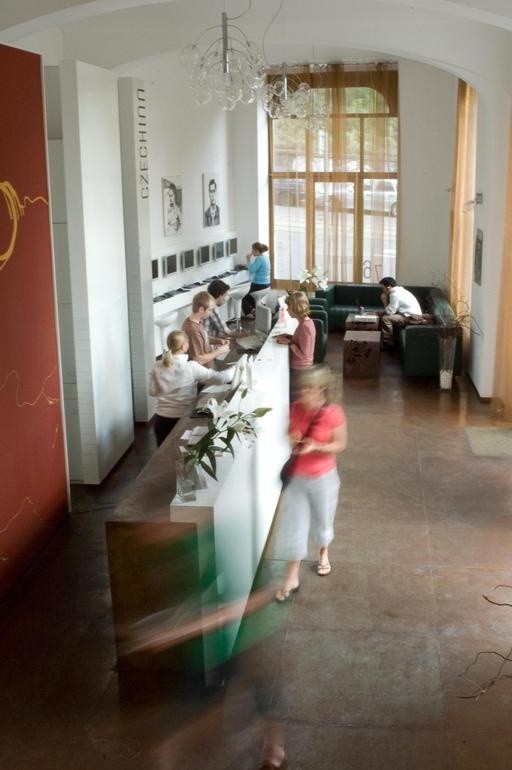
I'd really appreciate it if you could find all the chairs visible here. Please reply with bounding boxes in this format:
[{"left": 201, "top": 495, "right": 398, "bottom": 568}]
[
  {"left": 273, "top": 309, "right": 330, "bottom": 361},
  {"left": 283, "top": 295, "right": 328, "bottom": 311}
]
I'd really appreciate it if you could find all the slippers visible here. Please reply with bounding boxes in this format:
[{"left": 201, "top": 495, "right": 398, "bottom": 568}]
[
  {"left": 316, "top": 561, "right": 332, "bottom": 577},
  {"left": 277, "top": 584, "right": 300, "bottom": 603}
]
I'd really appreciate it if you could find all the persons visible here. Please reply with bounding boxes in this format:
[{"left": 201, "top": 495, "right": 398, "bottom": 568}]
[
  {"left": 379, "top": 277, "right": 423, "bottom": 347},
  {"left": 147, "top": 331, "right": 237, "bottom": 449},
  {"left": 242, "top": 243, "right": 271, "bottom": 316},
  {"left": 205, "top": 179, "right": 220, "bottom": 225},
  {"left": 271, "top": 291, "right": 316, "bottom": 370},
  {"left": 181, "top": 291, "right": 231, "bottom": 372},
  {"left": 165, "top": 184, "right": 182, "bottom": 234},
  {"left": 200, "top": 279, "right": 249, "bottom": 348},
  {"left": 275, "top": 365, "right": 347, "bottom": 602}
]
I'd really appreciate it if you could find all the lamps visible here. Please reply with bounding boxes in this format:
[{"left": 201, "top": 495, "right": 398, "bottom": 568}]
[
  {"left": 259, "top": 0, "right": 310, "bottom": 125},
  {"left": 306, "top": 0, "right": 330, "bottom": 132},
  {"left": 179, "top": 0, "right": 269, "bottom": 113},
  {"left": 461, "top": 190, "right": 485, "bottom": 214}
]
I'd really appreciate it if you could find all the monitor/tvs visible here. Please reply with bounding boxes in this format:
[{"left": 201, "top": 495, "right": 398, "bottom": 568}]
[
  {"left": 182, "top": 248, "right": 195, "bottom": 272},
  {"left": 255, "top": 304, "right": 272, "bottom": 338},
  {"left": 214, "top": 240, "right": 224, "bottom": 262},
  {"left": 164, "top": 254, "right": 177, "bottom": 278},
  {"left": 227, "top": 237, "right": 238, "bottom": 257},
  {"left": 152, "top": 259, "right": 158, "bottom": 280},
  {"left": 199, "top": 245, "right": 210, "bottom": 266}
]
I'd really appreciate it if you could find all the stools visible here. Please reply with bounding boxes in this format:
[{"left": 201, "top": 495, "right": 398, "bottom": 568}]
[
  {"left": 155, "top": 312, "right": 178, "bottom": 358},
  {"left": 230, "top": 285, "right": 251, "bottom": 332},
  {"left": 249, "top": 285, "right": 271, "bottom": 305}
]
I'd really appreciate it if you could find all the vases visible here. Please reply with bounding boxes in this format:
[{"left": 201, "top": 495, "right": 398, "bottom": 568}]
[
  {"left": 174, "top": 444, "right": 208, "bottom": 503},
  {"left": 439, "top": 335, "right": 456, "bottom": 392}
]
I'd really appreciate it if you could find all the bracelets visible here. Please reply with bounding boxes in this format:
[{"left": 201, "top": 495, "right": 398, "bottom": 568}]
[{"left": 288, "top": 341, "right": 295, "bottom": 345}]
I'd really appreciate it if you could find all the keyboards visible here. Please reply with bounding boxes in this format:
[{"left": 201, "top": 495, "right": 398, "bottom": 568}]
[
  {"left": 153, "top": 265, "right": 247, "bottom": 302},
  {"left": 236, "top": 335, "right": 262, "bottom": 350}
]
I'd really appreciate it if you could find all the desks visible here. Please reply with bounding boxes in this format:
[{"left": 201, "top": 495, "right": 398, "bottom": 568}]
[
  {"left": 345, "top": 313, "right": 380, "bottom": 331},
  {"left": 341, "top": 330, "right": 382, "bottom": 379}
]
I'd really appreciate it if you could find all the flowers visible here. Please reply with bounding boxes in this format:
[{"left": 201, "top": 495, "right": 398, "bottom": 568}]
[
  {"left": 188, "top": 349, "right": 273, "bottom": 484},
  {"left": 297, "top": 269, "right": 328, "bottom": 292}
]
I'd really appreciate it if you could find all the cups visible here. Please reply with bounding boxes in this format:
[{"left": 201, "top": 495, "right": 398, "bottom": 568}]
[{"left": 360, "top": 307, "right": 365, "bottom": 316}]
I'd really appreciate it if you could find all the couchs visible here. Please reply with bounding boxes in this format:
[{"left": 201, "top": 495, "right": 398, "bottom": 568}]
[{"left": 316, "top": 285, "right": 465, "bottom": 377}]
